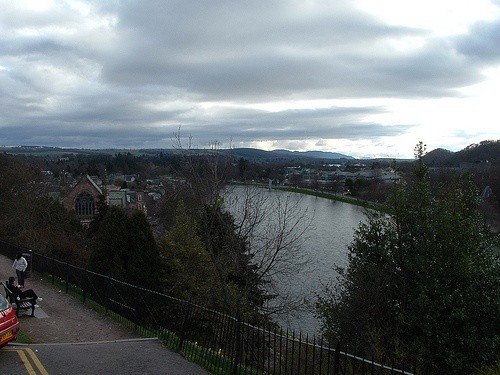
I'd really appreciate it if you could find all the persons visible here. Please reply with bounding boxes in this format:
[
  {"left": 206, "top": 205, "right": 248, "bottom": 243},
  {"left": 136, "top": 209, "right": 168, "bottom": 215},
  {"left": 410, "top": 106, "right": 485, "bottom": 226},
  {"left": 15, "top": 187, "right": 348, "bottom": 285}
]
[
  {"left": 11, "top": 251, "right": 28, "bottom": 287},
  {"left": 7, "top": 277, "right": 43, "bottom": 308}
]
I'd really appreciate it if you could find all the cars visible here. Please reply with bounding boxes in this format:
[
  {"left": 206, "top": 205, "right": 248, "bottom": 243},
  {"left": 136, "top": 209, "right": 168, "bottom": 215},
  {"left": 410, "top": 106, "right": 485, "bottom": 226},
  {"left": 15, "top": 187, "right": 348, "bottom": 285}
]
[{"left": 0, "top": 293, "right": 20, "bottom": 350}]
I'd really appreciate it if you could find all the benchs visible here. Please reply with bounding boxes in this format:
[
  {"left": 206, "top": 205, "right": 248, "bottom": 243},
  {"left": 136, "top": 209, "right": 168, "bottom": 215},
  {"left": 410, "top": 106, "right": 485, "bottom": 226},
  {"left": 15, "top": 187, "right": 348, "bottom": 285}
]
[{"left": 0, "top": 282, "right": 36, "bottom": 318}]
